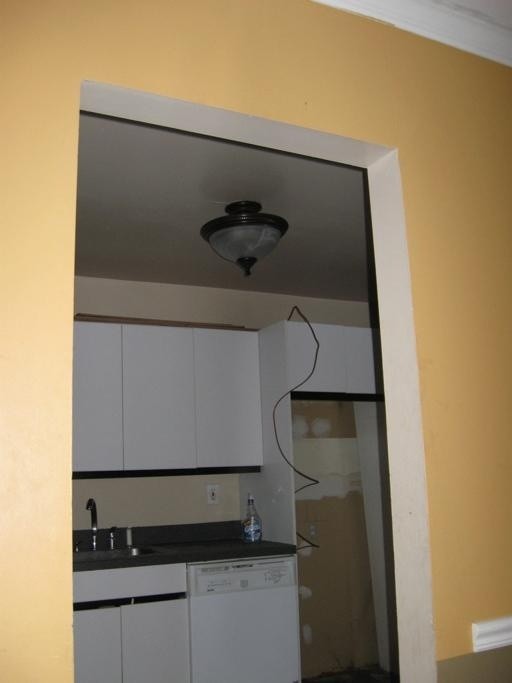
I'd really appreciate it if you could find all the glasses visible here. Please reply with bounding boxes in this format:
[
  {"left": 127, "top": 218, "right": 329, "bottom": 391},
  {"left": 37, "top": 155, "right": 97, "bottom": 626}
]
[{"left": 198, "top": 199, "right": 290, "bottom": 279}]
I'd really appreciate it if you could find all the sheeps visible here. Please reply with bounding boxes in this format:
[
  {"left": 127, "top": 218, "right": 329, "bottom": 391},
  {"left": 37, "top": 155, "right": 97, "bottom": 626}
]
[{"left": 84, "top": 497, "right": 99, "bottom": 550}]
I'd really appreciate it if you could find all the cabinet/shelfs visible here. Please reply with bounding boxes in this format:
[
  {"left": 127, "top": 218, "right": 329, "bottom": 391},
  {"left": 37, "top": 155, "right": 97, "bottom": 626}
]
[
  {"left": 75, "top": 318, "right": 123, "bottom": 479},
  {"left": 73, "top": 563, "right": 192, "bottom": 682},
  {"left": 242, "top": 492, "right": 262, "bottom": 542},
  {"left": 122, "top": 314, "right": 263, "bottom": 480}
]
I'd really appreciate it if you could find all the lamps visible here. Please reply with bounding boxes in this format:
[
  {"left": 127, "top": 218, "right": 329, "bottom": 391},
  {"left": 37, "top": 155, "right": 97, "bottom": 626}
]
[{"left": 198, "top": 199, "right": 290, "bottom": 279}]
